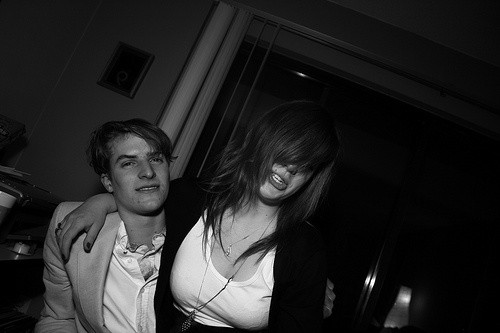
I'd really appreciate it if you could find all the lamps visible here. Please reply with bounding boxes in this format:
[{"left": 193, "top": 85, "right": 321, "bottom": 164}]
[{"left": 96, "top": 41, "right": 155, "bottom": 100}]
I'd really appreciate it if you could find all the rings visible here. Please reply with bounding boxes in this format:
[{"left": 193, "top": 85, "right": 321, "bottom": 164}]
[{"left": 57, "top": 223, "right": 63, "bottom": 230}]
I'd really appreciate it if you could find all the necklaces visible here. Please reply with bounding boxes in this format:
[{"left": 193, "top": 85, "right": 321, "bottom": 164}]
[
  {"left": 219, "top": 210, "right": 278, "bottom": 256},
  {"left": 182, "top": 205, "right": 280, "bottom": 332}
]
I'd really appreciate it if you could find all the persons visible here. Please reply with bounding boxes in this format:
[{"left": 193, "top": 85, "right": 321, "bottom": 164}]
[
  {"left": 31, "top": 116, "right": 336, "bottom": 332},
  {"left": 54, "top": 96, "right": 341, "bottom": 332}
]
